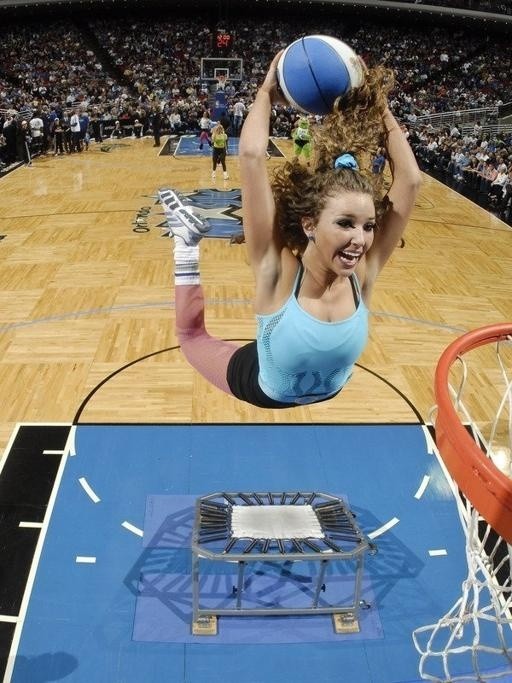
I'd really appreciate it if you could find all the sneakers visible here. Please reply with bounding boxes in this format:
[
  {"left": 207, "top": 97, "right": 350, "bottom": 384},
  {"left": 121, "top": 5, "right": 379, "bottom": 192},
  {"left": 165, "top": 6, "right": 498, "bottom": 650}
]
[
  {"left": 224, "top": 176, "right": 229, "bottom": 179},
  {"left": 212, "top": 173, "right": 216, "bottom": 178},
  {"left": 159, "top": 188, "right": 211, "bottom": 247}
]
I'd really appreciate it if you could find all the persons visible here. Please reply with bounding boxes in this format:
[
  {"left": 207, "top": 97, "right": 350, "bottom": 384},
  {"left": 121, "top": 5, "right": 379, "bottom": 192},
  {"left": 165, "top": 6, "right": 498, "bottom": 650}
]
[
  {"left": 156, "top": 46, "right": 422, "bottom": 410},
  {"left": 1, "top": 0, "right": 511, "bottom": 227}
]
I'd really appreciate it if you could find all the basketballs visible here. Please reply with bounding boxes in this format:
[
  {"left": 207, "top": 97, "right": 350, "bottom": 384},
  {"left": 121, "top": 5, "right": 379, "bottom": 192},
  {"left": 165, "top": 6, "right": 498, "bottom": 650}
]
[{"left": 277, "top": 34, "right": 364, "bottom": 115}]
